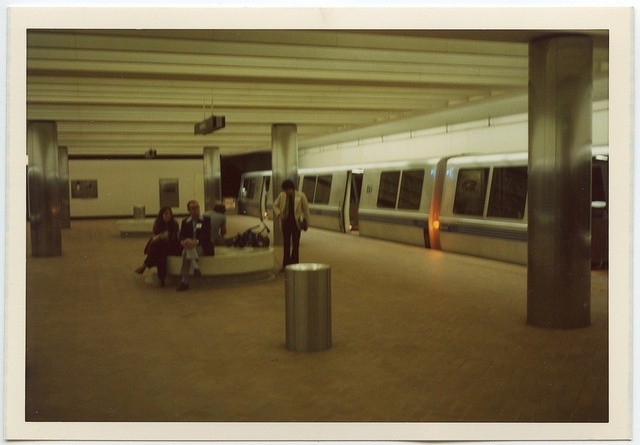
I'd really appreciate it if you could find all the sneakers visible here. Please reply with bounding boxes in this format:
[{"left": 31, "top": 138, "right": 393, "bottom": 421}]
[{"left": 136, "top": 267, "right": 145, "bottom": 274}]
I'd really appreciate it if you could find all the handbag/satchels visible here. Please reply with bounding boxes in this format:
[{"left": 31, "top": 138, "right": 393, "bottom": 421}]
[{"left": 302, "top": 217, "right": 307, "bottom": 231}]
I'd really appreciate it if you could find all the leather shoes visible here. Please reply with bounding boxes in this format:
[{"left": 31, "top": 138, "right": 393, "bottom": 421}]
[{"left": 193, "top": 268, "right": 201, "bottom": 276}]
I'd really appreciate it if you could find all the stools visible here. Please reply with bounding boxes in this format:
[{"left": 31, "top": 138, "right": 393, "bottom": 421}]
[
  {"left": 166, "top": 243, "right": 275, "bottom": 277},
  {"left": 118, "top": 220, "right": 156, "bottom": 233}
]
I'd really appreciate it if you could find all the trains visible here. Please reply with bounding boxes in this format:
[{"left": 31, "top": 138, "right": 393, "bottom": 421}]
[{"left": 238, "top": 146, "right": 607, "bottom": 268}]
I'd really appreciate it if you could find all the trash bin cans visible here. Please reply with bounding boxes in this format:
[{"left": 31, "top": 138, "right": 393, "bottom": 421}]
[
  {"left": 134, "top": 204, "right": 146, "bottom": 219},
  {"left": 285, "top": 262, "right": 332, "bottom": 352}
]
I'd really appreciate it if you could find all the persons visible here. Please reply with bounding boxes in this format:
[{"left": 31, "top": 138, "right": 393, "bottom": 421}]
[
  {"left": 237, "top": 187, "right": 248, "bottom": 215},
  {"left": 272, "top": 178, "right": 310, "bottom": 273},
  {"left": 176, "top": 199, "right": 215, "bottom": 291},
  {"left": 134, "top": 206, "right": 180, "bottom": 288},
  {"left": 205, "top": 203, "right": 227, "bottom": 246}
]
